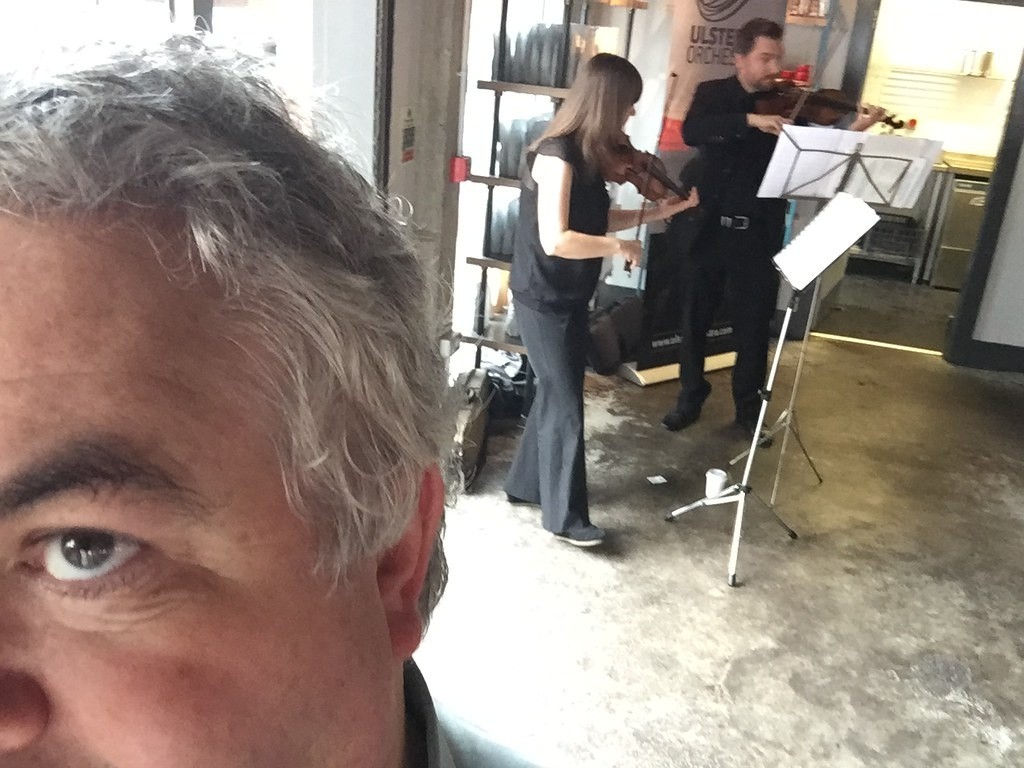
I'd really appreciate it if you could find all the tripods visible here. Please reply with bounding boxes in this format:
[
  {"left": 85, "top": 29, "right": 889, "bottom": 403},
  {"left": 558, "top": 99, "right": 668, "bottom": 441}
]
[{"left": 663, "top": 142, "right": 914, "bottom": 587}]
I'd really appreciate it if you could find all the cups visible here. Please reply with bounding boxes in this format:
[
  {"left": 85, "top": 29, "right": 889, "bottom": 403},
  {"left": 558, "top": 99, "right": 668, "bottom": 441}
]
[{"left": 704, "top": 467, "right": 728, "bottom": 498}]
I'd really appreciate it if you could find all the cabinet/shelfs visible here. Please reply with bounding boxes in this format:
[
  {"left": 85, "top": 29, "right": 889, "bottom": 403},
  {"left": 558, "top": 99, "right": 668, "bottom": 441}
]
[{"left": 460, "top": 0, "right": 649, "bottom": 412}]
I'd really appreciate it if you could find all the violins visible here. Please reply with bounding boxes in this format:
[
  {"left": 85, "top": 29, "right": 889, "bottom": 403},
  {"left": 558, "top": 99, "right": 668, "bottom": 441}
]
[
  {"left": 601, "top": 129, "right": 690, "bottom": 203},
  {"left": 753, "top": 79, "right": 904, "bottom": 130}
]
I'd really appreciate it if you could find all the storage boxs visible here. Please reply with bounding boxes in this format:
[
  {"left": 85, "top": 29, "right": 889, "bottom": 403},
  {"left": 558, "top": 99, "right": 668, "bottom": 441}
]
[{"left": 447, "top": 368, "right": 489, "bottom": 492}]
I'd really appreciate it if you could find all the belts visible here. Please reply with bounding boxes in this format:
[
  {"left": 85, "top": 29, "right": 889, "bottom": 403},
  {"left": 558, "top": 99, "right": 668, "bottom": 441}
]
[{"left": 698, "top": 210, "right": 771, "bottom": 235}]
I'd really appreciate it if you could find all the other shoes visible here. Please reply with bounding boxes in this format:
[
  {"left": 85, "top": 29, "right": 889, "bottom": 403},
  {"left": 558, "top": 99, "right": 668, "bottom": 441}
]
[
  {"left": 662, "top": 409, "right": 700, "bottom": 430},
  {"left": 507, "top": 493, "right": 538, "bottom": 507},
  {"left": 735, "top": 416, "right": 776, "bottom": 449},
  {"left": 554, "top": 521, "right": 605, "bottom": 547}
]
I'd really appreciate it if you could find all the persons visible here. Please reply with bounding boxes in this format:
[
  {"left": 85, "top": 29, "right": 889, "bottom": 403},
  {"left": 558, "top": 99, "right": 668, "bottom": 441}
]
[
  {"left": 502, "top": 53, "right": 699, "bottom": 546},
  {"left": 661, "top": 16, "right": 886, "bottom": 448},
  {"left": 0, "top": 37, "right": 535, "bottom": 768}
]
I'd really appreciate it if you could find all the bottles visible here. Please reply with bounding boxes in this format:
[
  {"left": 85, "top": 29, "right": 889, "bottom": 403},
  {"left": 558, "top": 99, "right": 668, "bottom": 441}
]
[
  {"left": 498, "top": 115, "right": 551, "bottom": 183},
  {"left": 490, "top": 197, "right": 521, "bottom": 256},
  {"left": 491, "top": 22, "right": 564, "bottom": 89}
]
